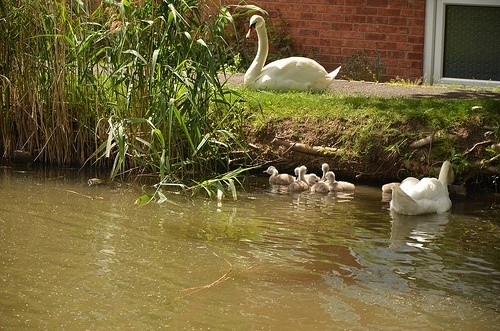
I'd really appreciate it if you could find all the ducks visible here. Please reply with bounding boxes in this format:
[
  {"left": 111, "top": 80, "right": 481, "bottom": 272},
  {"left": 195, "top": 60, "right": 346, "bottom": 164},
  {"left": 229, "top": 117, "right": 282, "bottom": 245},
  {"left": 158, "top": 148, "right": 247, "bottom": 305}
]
[{"left": 262, "top": 162, "right": 355, "bottom": 194}]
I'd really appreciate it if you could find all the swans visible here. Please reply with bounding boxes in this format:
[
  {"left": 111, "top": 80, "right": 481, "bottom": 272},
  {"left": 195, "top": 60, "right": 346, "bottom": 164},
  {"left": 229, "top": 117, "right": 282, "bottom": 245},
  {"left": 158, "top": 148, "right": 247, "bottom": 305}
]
[
  {"left": 389, "top": 160, "right": 456, "bottom": 215},
  {"left": 242, "top": 14, "right": 343, "bottom": 94}
]
